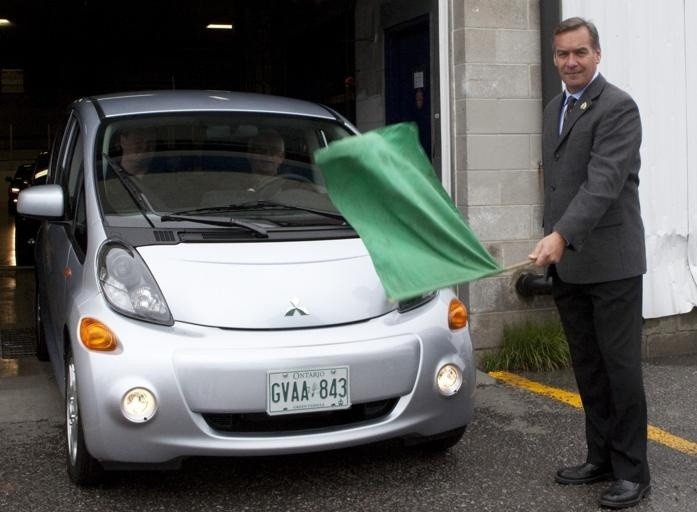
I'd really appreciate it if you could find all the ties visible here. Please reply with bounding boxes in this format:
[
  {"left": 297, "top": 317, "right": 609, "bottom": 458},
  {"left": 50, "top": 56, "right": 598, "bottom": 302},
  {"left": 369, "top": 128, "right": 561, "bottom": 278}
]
[{"left": 563, "top": 96, "right": 577, "bottom": 130}]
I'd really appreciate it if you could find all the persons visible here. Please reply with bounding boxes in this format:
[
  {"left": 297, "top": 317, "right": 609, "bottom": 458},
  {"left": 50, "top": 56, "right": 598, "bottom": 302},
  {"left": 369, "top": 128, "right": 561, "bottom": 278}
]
[
  {"left": 218, "top": 130, "right": 326, "bottom": 198},
  {"left": 101, "top": 125, "right": 197, "bottom": 214},
  {"left": 529, "top": 18, "right": 651, "bottom": 508}
]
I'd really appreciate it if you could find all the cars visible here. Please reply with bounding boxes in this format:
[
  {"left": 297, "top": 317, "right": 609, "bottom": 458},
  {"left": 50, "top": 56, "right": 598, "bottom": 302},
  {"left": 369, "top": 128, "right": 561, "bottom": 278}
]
[{"left": 16, "top": 85, "right": 477, "bottom": 484}]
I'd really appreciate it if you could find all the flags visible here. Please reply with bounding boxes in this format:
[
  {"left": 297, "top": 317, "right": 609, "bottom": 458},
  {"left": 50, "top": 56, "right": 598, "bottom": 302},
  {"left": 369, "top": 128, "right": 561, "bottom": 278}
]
[{"left": 313, "top": 121, "right": 505, "bottom": 303}]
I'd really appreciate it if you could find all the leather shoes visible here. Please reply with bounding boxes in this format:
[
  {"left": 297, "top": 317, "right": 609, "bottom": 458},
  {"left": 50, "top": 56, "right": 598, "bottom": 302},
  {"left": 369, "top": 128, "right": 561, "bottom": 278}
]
[
  {"left": 599, "top": 478, "right": 651, "bottom": 508},
  {"left": 554, "top": 462, "right": 613, "bottom": 485}
]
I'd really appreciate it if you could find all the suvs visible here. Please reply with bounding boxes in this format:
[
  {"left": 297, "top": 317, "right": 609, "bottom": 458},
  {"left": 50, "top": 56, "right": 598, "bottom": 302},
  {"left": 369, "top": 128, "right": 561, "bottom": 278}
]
[
  {"left": 14, "top": 149, "right": 56, "bottom": 268},
  {"left": 4, "top": 162, "right": 34, "bottom": 217}
]
